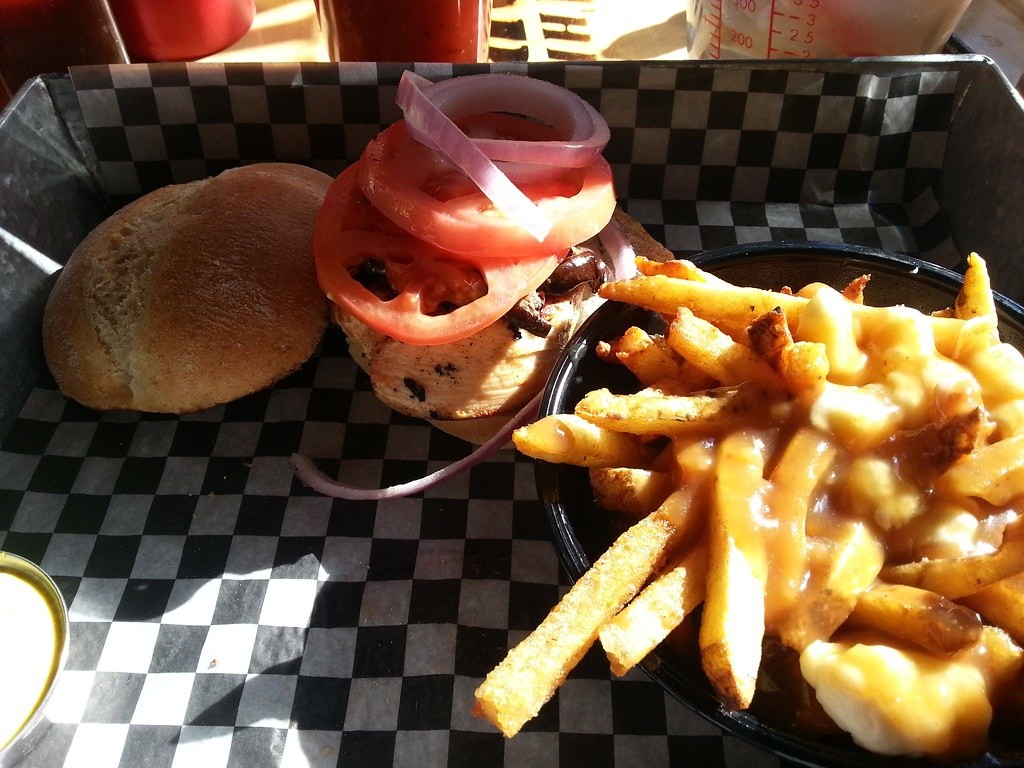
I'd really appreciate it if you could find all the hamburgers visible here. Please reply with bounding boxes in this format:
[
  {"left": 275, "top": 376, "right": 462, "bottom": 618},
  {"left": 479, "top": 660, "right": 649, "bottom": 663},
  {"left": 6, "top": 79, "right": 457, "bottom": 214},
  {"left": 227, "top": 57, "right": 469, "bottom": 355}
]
[{"left": 42, "top": 68, "right": 672, "bottom": 501}]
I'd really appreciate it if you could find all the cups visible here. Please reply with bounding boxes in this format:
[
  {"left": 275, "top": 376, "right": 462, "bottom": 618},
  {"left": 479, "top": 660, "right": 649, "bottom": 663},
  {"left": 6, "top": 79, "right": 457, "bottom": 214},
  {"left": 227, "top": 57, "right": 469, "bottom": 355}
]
[
  {"left": 312, "top": 0, "right": 491, "bottom": 65},
  {"left": 1, "top": 0, "right": 130, "bottom": 118},
  {"left": 685, "top": 0, "right": 973, "bottom": 60},
  {"left": 108, "top": 0, "right": 254, "bottom": 64}
]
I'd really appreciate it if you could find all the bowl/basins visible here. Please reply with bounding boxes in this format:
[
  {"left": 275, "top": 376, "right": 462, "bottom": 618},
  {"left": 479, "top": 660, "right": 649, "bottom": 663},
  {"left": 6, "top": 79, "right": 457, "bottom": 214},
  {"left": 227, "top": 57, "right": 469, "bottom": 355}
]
[
  {"left": 535, "top": 238, "right": 1024, "bottom": 768},
  {"left": 0, "top": 549, "right": 70, "bottom": 762}
]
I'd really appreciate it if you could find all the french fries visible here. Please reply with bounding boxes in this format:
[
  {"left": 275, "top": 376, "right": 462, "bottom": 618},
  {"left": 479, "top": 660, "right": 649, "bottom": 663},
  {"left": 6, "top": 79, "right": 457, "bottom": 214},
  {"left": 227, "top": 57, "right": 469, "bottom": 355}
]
[{"left": 468, "top": 254, "right": 1023, "bottom": 739}]
